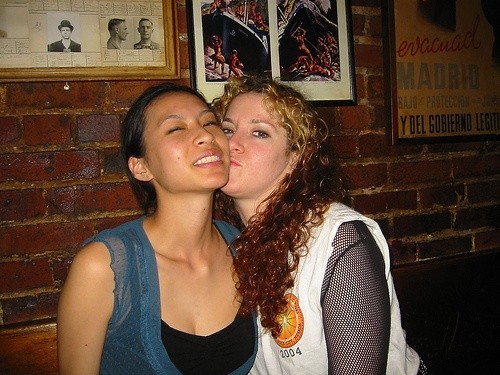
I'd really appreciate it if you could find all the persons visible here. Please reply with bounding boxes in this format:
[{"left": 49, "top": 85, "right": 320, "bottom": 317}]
[
  {"left": 48, "top": 19, "right": 82, "bottom": 52},
  {"left": 133, "top": 18, "right": 161, "bottom": 48},
  {"left": 59, "top": 83, "right": 263, "bottom": 374},
  {"left": 107, "top": 18, "right": 128, "bottom": 49},
  {"left": 208, "top": 77, "right": 430, "bottom": 375}
]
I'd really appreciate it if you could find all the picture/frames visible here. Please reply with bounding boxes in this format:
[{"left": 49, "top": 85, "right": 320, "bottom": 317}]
[
  {"left": 0, "top": 0, "right": 180, "bottom": 82},
  {"left": 382, "top": 0, "right": 500, "bottom": 146},
  {"left": 185, "top": 0, "right": 357, "bottom": 107}
]
